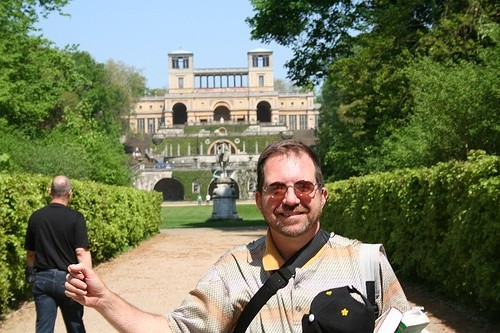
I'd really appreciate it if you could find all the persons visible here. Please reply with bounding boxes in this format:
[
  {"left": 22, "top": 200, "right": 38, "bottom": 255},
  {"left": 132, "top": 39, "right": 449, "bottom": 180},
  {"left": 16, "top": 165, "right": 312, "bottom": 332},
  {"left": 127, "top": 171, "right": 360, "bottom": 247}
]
[
  {"left": 206, "top": 194, "right": 210, "bottom": 205},
  {"left": 64, "top": 140, "right": 432, "bottom": 333},
  {"left": 197, "top": 194, "right": 202, "bottom": 206},
  {"left": 25, "top": 176, "right": 92, "bottom": 333}
]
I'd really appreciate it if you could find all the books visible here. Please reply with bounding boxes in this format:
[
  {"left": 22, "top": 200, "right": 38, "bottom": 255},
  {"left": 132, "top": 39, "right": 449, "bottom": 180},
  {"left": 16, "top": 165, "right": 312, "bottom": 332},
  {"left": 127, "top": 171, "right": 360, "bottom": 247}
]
[{"left": 373, "top": 305, "right": 431, "bottom": 333}]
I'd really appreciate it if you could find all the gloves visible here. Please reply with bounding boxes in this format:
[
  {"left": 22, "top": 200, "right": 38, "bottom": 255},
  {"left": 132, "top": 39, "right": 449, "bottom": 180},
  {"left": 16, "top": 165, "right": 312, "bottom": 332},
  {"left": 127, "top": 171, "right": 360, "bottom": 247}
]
[{"left": 25, "top": 264, "right": 35, "bottom": 283}]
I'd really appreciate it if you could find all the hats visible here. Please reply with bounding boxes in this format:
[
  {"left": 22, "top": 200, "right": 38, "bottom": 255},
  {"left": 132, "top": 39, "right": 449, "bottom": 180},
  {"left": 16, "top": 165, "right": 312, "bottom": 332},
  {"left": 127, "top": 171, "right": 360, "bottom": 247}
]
[{"left": 301, "top": 284, "right": 376, "bottom": 333}]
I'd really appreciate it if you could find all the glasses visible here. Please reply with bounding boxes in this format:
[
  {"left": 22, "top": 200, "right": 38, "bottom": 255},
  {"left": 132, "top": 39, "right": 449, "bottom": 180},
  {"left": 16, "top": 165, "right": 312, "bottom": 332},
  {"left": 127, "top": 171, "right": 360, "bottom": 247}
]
[{"left": 262, "top": 179, "right": 320, "bottom": 196}]
